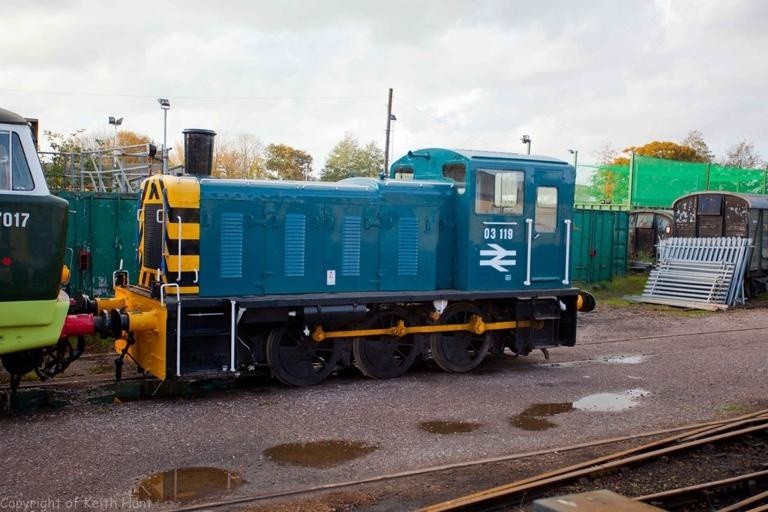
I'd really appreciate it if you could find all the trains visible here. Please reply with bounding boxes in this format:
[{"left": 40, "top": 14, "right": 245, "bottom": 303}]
[{"left": 1, "top": 109, "right": 597, "bottom": 387}]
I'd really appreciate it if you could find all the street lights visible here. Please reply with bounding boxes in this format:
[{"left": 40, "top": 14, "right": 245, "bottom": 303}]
[
  {"left": 568, "top": 149, "right": 577, "bottom": 172},
  {"left": 520, "top": 135, "right": 530, "bottom": 155},
  {"left": 108, "top": 115, "right": 123, "bottom": 194},
  {"left": 156, "top": 97, "right": 170, "bottom": 176}
]
